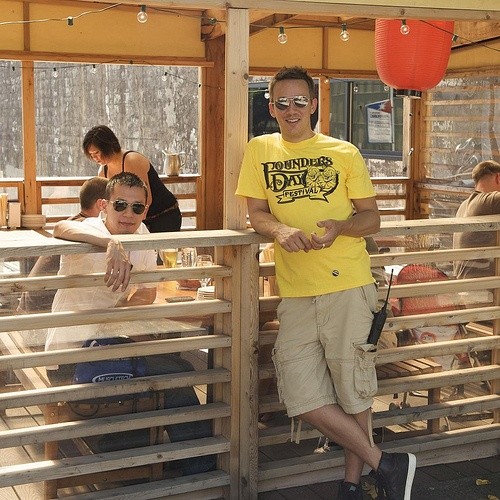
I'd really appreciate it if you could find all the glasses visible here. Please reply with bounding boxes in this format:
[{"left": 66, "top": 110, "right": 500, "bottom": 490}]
[
  {"left": 271, "top": 95, "right": 313, "bottom": 110},
  {"left": 105, "top": 199, "right": 145, "bottom": 215}
]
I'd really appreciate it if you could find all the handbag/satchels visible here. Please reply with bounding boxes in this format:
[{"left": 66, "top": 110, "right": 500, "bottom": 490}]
[{"left": 69, "top": 334, "right": 153, "bottom": 404}]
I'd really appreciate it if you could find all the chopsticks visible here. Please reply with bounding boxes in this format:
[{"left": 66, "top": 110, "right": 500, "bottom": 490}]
[{"left": 262, "top": 248, "right": 275, "bottom": 262}]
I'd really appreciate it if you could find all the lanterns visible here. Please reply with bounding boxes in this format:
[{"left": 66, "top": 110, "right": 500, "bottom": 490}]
[{"left": 374, "top": 19, "right": 455, "bottom": 98}]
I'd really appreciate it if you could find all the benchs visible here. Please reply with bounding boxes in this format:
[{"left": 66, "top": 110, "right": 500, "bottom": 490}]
[{"left": 0, "top": 264, "right": 500, "bottom": 500}]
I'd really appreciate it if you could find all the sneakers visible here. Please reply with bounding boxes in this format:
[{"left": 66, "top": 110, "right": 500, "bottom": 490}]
[
  {"left": 369, "top": 451, "right": 417, "bottom": 500},
  {"left": 338, "top": 480, "right": 363, "bottom": 500}
]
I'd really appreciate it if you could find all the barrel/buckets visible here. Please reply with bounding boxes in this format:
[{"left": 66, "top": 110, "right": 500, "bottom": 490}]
[{"left": 415, "top": 326, "right": 459, "bottom": 371}]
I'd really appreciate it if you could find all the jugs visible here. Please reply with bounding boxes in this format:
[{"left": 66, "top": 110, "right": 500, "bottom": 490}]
[{"left": 162, "top": 149, "right": 187, "bottom": 176}]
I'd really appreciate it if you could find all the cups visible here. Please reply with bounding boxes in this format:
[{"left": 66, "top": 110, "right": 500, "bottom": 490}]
[
  {"left": 158, "top": 247, "right": 213, "bottom": 287},
  {"left": 269, "top": 276, "right": 281, "bottom": 296},
  {"left": 259, "top": 276, "right": 264, "bottom": 297}
]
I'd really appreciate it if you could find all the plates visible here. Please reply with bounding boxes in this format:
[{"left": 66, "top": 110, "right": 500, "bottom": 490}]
[{"left": 197, "top": 286, "right": 215, "bottom": 300}]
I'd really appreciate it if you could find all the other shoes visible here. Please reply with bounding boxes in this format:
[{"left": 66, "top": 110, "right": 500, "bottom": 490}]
[{"left": 314, "top": 437, "right": 342, "bottom": 454}]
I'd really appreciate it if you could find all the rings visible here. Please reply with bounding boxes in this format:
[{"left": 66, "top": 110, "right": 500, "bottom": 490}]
[{"left": 322, "top": 243, "right": 325, "bottom": 249}]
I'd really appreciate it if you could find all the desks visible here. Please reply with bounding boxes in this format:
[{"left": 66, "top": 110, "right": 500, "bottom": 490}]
[
  {"left": 0, "top": 225, "right": 54, "bottom": 308},
  {"left": 125, "top": 260, "right": 282, "bottom": 473},
  {"left": 383, "top": 262, "right": 493, "bottom": 426}
]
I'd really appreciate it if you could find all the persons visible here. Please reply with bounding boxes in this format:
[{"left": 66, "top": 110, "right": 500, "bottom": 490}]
[
  {"left": 235, "top": 67, "right": 416, "bottom": 500},
  {"left": 453, "top": 160, "right": 500, "bottom": 328},
  {"left": 15, "top": 124, "right": 218, "bottom": 473}
]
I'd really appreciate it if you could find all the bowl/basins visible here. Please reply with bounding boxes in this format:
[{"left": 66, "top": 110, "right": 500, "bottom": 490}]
[{"left": 176, "top": 279, "right": 203, "bottom": 290}]
